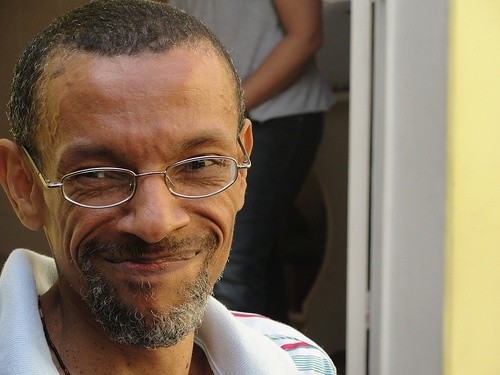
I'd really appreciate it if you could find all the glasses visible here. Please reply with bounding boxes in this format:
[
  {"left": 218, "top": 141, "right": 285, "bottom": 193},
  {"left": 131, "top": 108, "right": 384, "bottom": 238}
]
[{"left": 22, "top": 131, "right": 252, "bottom": 209}]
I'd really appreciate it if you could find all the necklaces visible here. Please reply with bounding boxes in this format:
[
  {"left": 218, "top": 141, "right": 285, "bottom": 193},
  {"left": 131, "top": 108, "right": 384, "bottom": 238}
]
[{"left": 36, "top": 292, "right": 80, "bottom": 375}]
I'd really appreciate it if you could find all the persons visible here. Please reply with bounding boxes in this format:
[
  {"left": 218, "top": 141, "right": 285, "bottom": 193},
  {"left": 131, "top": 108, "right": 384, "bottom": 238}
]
[
  {"left": 1, "top": 0, "right": 337, "bottom": 375},
  {"left": 164, "top": 1, "right": 330, "bottom": 325}
]
